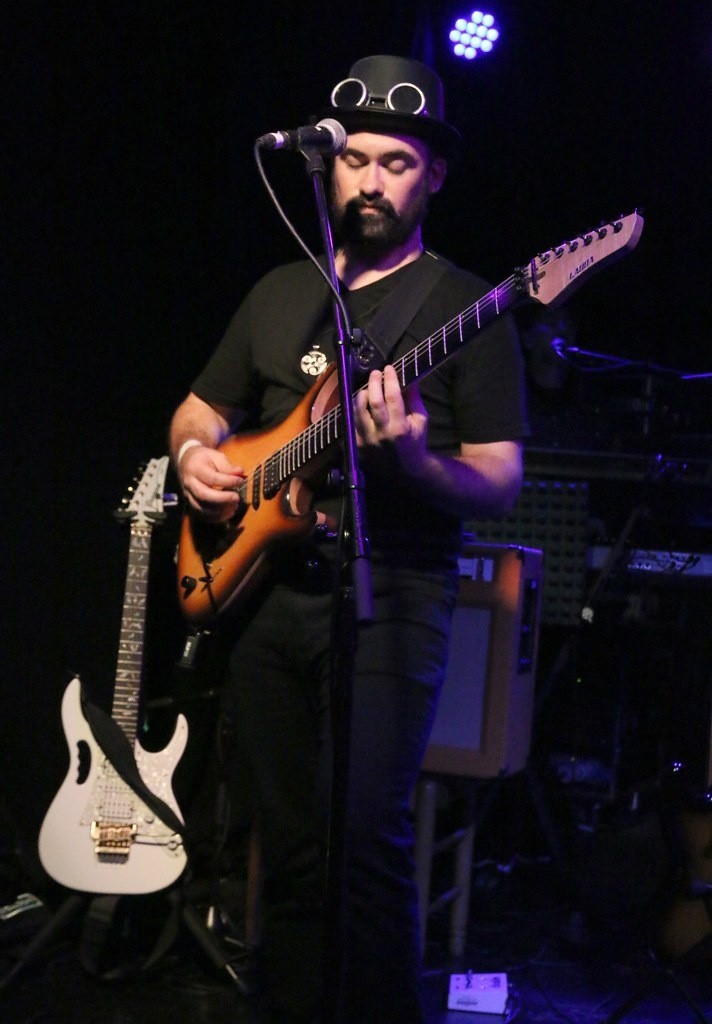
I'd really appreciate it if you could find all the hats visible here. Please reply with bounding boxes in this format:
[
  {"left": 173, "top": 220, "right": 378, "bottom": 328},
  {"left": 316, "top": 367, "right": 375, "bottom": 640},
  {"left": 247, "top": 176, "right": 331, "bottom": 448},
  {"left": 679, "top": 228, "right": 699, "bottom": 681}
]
[{"left": 309, "top": 55, "right": 464, "bottom": 157}]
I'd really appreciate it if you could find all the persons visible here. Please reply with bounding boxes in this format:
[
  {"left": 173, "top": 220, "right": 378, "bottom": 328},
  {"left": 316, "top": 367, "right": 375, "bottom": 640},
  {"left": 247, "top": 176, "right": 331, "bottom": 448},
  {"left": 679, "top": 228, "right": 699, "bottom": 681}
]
[{"left": 168, "top": 54, "right": 528, "bottom": 1024}]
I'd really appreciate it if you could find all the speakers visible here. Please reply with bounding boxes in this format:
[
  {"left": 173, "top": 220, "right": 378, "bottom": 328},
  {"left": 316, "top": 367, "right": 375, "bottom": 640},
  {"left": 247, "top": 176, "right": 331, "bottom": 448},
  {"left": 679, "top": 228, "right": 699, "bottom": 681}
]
[{"left": 418, "top": 543, "right": 547, "bottom": 782}]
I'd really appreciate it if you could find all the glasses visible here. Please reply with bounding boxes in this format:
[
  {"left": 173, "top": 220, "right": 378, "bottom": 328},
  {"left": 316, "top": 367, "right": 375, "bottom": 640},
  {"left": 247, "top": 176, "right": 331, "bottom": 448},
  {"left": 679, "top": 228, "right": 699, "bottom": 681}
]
[{"left": 330, "top": 76, "right": 429, "bottom": 115}]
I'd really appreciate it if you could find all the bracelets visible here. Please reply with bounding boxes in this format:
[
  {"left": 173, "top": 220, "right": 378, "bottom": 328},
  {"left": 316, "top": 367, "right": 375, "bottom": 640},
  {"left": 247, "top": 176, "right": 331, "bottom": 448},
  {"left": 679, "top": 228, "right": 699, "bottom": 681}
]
[{"left": 178, "top": 439, "right": 201, "bottom": 464}]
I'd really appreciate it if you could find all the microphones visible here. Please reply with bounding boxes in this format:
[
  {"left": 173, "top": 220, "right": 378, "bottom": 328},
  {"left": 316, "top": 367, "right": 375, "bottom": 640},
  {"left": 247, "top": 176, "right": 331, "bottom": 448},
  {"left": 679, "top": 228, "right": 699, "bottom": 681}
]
[
  {"left": 257, "top": 116, "right": 347, "bottom": 157},
  {"left": 550, "top": 337, "right": 566, "bottom": 353}
]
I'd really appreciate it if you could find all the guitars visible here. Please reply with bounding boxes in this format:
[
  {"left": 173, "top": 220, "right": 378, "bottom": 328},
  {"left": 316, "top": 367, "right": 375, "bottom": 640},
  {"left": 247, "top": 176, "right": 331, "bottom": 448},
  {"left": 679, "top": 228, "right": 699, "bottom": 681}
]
[
  {"left": 177, "top": 212, "right": 645, "bottom": 619},
  {"left": 36, "top": 455, "right": 190, "bottom": 894}
]
